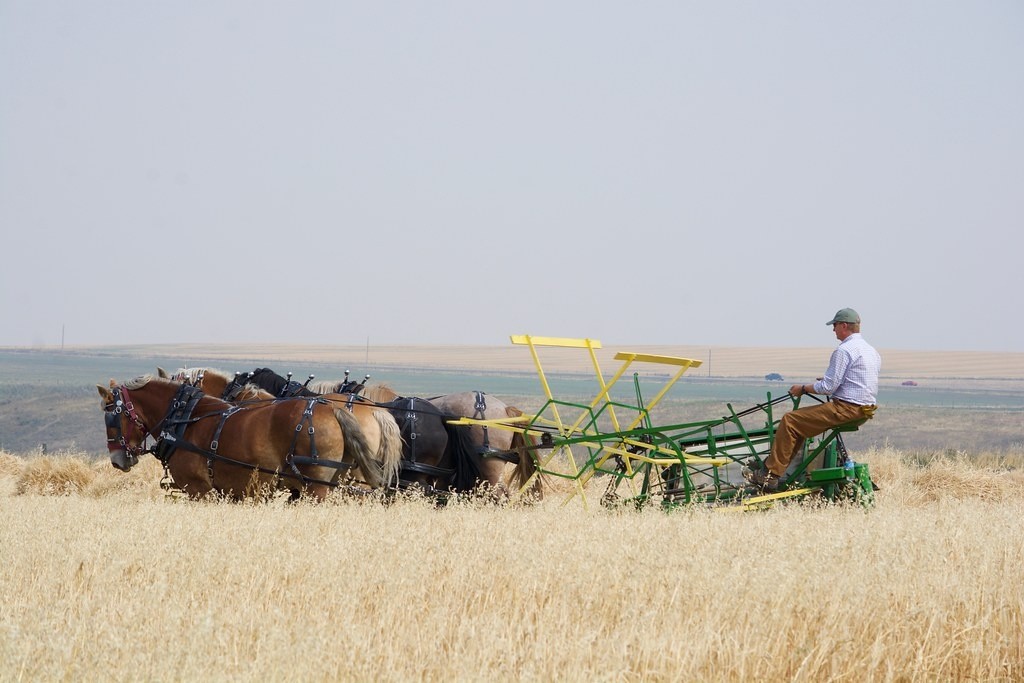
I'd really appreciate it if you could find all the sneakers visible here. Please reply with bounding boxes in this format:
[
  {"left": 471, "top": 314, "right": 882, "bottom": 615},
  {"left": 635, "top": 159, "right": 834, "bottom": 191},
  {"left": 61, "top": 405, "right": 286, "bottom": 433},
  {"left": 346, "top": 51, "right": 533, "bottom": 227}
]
[{"left": 742, "top": 467, "right": 778, "bottom": 489}]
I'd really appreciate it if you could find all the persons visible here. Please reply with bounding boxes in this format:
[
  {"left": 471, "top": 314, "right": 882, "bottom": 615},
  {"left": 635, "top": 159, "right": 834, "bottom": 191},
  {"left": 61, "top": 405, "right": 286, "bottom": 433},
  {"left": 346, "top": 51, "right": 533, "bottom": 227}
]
[{"left": 740, "top": 307, "right": 882, "bottom": 492}]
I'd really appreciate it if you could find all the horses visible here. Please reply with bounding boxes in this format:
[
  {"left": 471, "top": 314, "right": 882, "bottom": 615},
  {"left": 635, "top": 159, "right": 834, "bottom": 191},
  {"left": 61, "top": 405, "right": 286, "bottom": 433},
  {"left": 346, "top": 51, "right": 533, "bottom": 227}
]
[{"left": 93, "top": 364, "right": 559, "bottom": 509}]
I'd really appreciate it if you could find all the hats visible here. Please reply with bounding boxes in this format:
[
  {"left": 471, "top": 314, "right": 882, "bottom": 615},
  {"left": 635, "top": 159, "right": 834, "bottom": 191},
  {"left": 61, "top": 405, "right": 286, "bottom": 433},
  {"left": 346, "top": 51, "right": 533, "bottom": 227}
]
[{"left": 826, "top": 308, "right": 861, "bottom": 325}]
